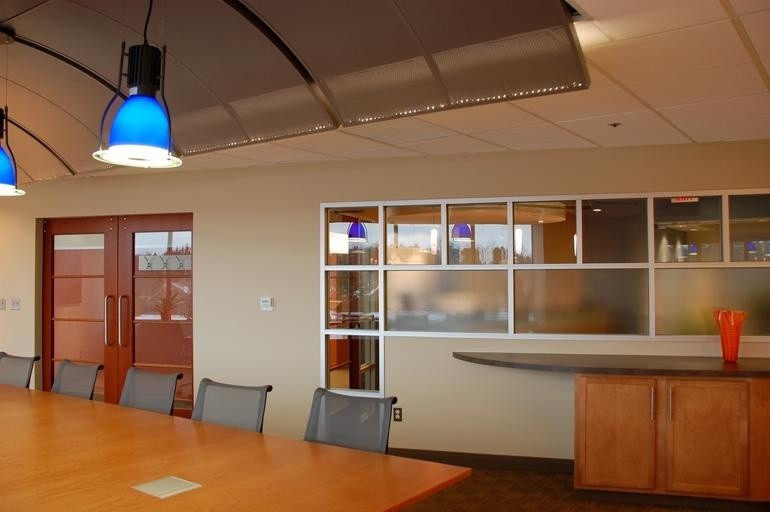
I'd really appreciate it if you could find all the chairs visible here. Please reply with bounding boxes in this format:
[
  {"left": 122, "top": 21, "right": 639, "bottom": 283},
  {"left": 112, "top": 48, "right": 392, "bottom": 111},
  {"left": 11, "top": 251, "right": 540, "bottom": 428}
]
[
  {"left": 190, "top": 377, "right": 273, "bottom": 433},
  {"left": 51, "top": 359, "right": 105, "bottom": 400},
  {"left": 0, "top": 351, "right": 41, "bottom": 389},
  {"left": 118, "top": 367, "right": 184, "bottom": 415},
  {"left": 303, "top": 387, "right": 397, "bottom": 453}
]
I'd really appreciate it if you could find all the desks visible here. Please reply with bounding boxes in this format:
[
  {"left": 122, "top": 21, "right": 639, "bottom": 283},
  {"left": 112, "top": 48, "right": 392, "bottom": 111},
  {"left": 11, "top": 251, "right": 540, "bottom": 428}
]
[{"left": 0, "top": 382, "right": 475, "bottom": 511}]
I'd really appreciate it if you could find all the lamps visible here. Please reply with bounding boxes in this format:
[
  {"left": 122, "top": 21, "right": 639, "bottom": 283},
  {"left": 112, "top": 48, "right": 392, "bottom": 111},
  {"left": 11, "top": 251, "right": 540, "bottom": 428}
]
[
  {"left": 347, "top": 216, "right": 366, "bottom": 239},
  {"left": 0, "top": 24, "right": 26, "bottom": 197},
  {"left": 746, "top": 241, "right": 757, "bottom": 253},
  {"left": 688, "top": 244, "right": 699, "bottom": 255},
  {"left": 92, "top": 1, "right": 185, "bottom": 169},
  {"left": 451, "top": 223, "right": 473, "bottom": 241}
]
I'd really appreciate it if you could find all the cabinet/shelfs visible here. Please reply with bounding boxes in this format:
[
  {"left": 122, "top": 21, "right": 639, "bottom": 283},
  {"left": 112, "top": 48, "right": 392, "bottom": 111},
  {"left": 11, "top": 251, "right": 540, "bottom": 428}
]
[{"left": 574, "top": 373, "right": 748, "bottom": 501}]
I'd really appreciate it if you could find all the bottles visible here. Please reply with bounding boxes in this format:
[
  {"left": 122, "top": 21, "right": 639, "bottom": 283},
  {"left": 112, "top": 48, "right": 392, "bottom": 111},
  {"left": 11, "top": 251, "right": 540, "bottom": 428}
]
[{"left": 656, "top": 228, "right": 701, "bottom": 264}]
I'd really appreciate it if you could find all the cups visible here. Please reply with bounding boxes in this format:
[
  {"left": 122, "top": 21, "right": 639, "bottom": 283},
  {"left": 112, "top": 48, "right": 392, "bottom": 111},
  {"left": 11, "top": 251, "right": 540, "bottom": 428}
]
[
  {"left": 720, "top": 361, "right": 738, "bottom": 372},
  {"left": 714, "top": 309, "right": 751, "bottom": 364},
  {"left": 730, "top": 239, "right": 770, "bottom": 263}
]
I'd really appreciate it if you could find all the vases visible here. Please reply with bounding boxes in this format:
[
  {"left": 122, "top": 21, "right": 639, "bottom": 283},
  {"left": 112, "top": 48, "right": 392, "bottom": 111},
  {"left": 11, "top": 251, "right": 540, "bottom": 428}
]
[{"left": 712, "top": 308, "right": 748, "bottom": 362}]
